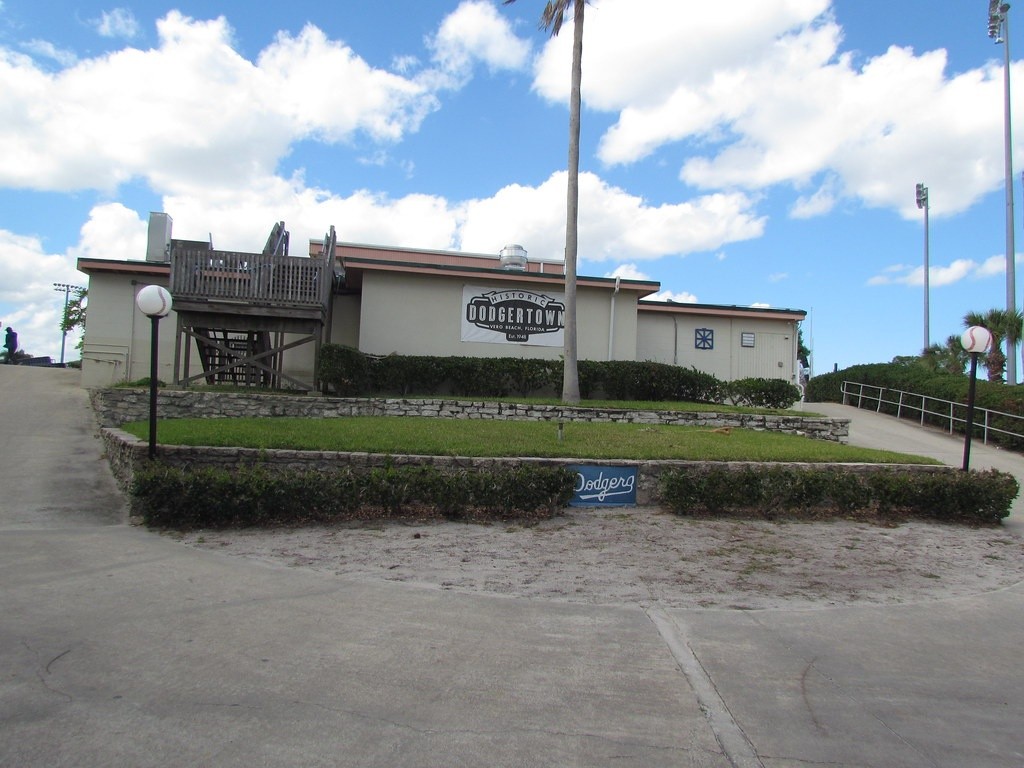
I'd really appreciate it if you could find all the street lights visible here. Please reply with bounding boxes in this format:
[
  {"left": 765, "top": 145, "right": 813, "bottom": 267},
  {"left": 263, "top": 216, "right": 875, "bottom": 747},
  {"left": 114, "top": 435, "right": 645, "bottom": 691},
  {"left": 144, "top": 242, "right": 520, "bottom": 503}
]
[
  {"left": 960, "top": 326, "right": 993, "bottom": 471},
  {"left": 916, "top": 182, "right": 931, "bottom": 352},
  {"left": 53, "top": 282, "right": 86, "bottom": 364},
  {"left": 987, "top": 0, "right": 1017, "bottom": 387},
  {"left": 136, "top": 284, "right": 173, "bottom": 459}
]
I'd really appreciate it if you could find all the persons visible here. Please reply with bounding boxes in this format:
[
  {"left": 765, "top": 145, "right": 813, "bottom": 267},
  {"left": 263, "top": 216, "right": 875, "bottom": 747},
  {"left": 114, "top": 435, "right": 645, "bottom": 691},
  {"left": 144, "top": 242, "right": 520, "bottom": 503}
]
[{"left": 3, "top": 327, "right": 19, "bottom": 365}]
